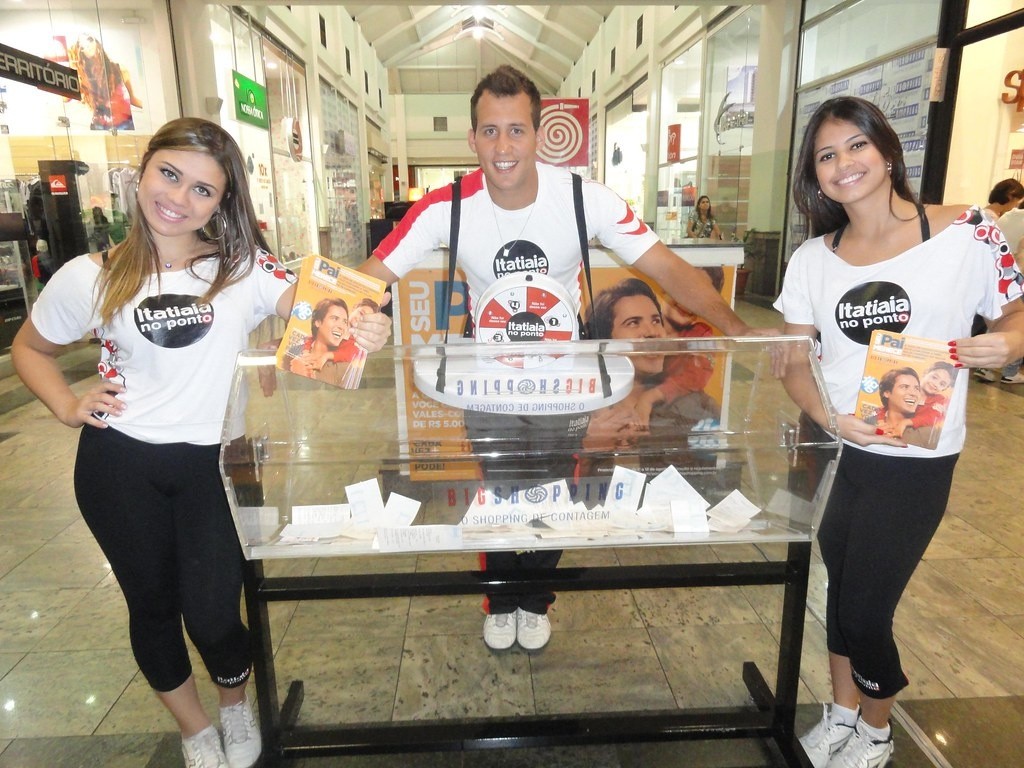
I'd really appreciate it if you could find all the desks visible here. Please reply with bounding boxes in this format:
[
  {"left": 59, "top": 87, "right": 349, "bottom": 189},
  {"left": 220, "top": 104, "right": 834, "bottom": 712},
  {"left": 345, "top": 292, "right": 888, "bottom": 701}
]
[{"left": 661, "top": 238, "right": 746, "bottom": 312}]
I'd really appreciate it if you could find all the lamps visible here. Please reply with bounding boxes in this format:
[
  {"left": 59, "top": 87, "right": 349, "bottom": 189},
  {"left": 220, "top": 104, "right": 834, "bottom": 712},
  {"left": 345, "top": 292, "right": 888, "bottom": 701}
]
[{"left": 205, "top": 96, "right": 223, "bottom": 115}]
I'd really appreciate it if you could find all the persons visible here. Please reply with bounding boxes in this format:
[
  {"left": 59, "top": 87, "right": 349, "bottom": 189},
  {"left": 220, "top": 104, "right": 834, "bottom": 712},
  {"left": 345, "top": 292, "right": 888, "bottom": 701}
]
[
  {"left": 573, "top": 277, "right": 723, "bottom": 479},
  {"left": 610, "top": 266, "right": 726, "bottom": 437},
  {"left": 972, "top": 207, "right": 1024, "bottom": 385},
  {"left": 10, "top": 117, "right": 391, "bottom": 768},
  {"left": 981, "top": 177, "right": 1024, "bottom": 222},
  {"left": 302, "top": 297, "right": 381, "bottom": 370},
  {"left": 26, "top": 198, "right": 53, "bottom": 285},
  {"left": 864, "top": 363, "right": 956, "bottom": 435},
  {"left": 772, "top": 96, "right": 1023, "bottom": 767},
  {"left": 259, "top": 64, "right": 791, "bottom": 652},
  {"left": 282, "top": 297, "right": 350, "bottom": 389},
  {"left": 88, "top": 205, "right": 110, "bottom": 251},
  {"left": 686, "top": 195, "right": 722, "bottom": 240}
]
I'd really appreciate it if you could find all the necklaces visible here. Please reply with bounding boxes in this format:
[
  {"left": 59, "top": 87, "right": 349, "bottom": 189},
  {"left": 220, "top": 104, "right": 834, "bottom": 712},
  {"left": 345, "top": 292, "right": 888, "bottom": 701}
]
[
  {"left": 486, "top": 177, "right": 541, "bottom": 257},
  {"left": 157, "top": 256, "right": 181, "bottom": 270}
]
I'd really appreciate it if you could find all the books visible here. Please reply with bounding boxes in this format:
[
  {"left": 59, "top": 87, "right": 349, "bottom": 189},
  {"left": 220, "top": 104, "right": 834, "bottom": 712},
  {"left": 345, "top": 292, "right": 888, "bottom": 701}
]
[{"left": 274, "top": 254, "right": 388, "bottom": 390}]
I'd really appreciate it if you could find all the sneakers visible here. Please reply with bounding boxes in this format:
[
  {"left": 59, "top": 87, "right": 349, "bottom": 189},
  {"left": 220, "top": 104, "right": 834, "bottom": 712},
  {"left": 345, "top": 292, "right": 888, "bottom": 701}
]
[
  {"left": 482, "top": 609, "right": 516, "bottom": 650},
  {"left": 798, "top": 702, "right": 858, "bottom": 768},
  {"left": 517, "top": 607, "right": 552, "bottom": 651},
  {"left": 973, "top": 366, "right": 997, "bottom": 381},
  {"left": 181, "top": 725, "right": 228, "bottom": 768},
  {"left": 218, "top": 693, "right": 262, "bottom": 768},
  {"left": 822, "top": 720, "right": 892, "bottom": 768},
  {"left": 1001, "top": 371, "right": 1024, "bottom": 384}
]
